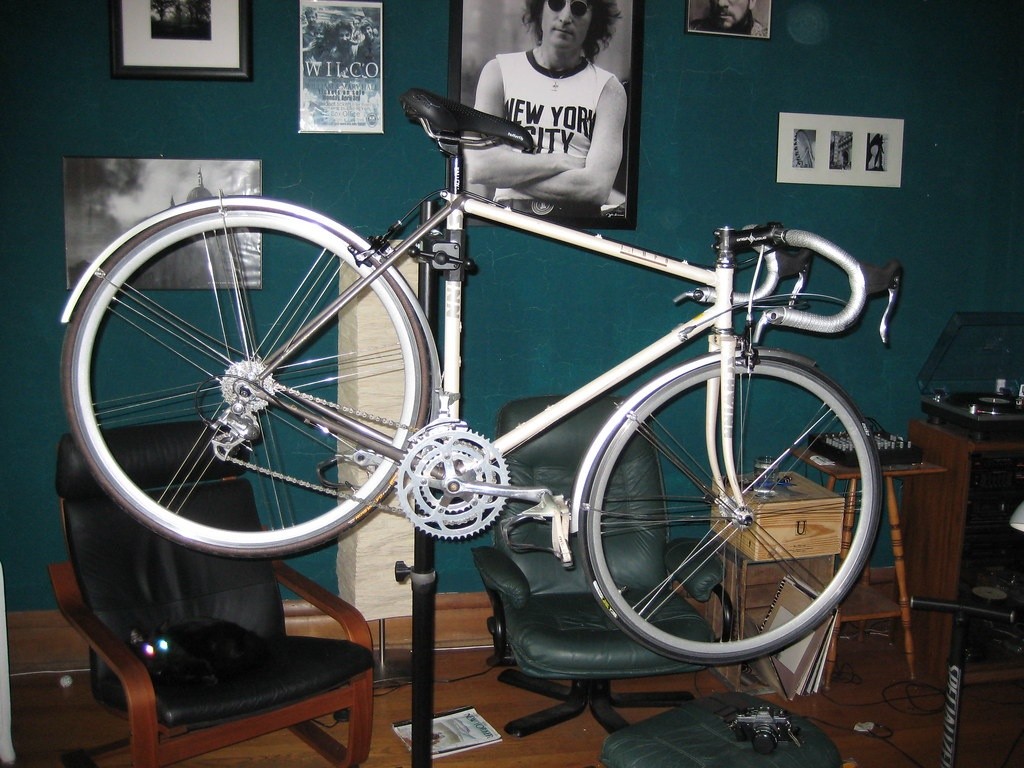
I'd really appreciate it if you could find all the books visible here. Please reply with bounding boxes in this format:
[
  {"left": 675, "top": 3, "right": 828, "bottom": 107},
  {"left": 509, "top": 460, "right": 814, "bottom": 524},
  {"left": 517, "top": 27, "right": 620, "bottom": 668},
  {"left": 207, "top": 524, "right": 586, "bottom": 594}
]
[
  {"left": 390, "top": 705, "right": 505, "bottom": 762},
  {"left": 742, "top": 570, "right": 845, "bottom": 703}
]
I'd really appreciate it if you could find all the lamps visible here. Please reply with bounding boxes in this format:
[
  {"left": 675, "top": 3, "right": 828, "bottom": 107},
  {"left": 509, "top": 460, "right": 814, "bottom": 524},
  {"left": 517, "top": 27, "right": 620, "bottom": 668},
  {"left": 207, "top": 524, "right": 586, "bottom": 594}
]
[{"left": 336, "top": 239, "right": 417, "bottom": 687}]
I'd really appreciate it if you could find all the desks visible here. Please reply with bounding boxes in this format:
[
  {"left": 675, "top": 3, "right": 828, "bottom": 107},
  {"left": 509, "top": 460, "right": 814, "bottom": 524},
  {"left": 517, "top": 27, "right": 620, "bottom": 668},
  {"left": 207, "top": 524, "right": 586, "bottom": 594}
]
[{"left": 790, "top": 447, "right": 949, "bottom": 692}]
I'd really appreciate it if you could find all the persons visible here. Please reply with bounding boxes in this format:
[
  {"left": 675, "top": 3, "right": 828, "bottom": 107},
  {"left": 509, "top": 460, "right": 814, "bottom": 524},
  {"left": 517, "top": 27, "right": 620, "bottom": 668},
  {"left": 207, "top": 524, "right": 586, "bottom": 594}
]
[
  {"left": 302, "top": 7, "right": 379, "bottom": 78},
  {"left": 459, "top": 0, "right": 627, "bottom": 217},
  {"left": 688, "top": 0, "right": 767, "bottom": 37}
]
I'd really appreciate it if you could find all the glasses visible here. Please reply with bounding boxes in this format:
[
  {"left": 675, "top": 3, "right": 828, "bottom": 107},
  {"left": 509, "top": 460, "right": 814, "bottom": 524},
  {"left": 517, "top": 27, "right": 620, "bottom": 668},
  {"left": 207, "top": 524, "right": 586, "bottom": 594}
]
[{"left": 548, "top": 0, "right": 594, "bottom": 17}]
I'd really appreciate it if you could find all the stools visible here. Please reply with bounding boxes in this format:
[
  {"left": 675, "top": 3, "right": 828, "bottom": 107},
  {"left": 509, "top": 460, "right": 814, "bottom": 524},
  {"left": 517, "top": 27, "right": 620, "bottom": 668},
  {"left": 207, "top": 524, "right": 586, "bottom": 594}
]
[{"left": 598, "top": 689, "right": 844, "bottom": 768}]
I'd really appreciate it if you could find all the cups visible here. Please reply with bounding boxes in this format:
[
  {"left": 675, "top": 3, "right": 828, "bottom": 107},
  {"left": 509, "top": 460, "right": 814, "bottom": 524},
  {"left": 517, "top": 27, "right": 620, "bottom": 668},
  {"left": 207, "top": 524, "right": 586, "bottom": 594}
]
[{"left": 754, "top": 457, "right": 778, "bottom": 496}]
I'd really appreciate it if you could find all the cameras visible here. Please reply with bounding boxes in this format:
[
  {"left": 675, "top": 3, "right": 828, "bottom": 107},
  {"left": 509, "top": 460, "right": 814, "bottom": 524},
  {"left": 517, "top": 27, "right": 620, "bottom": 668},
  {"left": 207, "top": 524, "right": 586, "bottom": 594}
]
[{"left": 729, "top": 707, "right": 794, "bottom": 755}]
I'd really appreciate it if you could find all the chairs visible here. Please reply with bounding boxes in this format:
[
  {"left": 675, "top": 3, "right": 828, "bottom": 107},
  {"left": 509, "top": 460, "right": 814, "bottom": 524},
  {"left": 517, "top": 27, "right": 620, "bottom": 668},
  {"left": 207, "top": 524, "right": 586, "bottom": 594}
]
[
  {"left": 48, "top": 420, "right": 376, "bottom": 768},
  {"left": 469, "top": 395, "right": 735, "bottom": 738}
]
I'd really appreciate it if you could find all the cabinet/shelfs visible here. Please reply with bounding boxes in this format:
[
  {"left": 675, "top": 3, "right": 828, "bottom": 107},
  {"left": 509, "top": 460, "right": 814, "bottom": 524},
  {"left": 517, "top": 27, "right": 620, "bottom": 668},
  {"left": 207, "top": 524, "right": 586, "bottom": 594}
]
[{"left": 890, "top": 418, "right": 1023, "bottom": 687}]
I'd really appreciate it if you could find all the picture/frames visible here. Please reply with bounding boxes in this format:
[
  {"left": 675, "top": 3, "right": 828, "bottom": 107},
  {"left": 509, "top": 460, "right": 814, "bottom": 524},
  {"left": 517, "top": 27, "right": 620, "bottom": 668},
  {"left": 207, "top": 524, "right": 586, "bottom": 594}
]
[
  {"left": 685, "top": 0, "right": 772, "bottom": 41},
  {"left": 775, "top": 111, "right": 904, "bottom": 189},
  {"left": 106, "top": 0, "right": 253, "bottom": 82},
  {"left": 445, "top": 0, "right": 645, "bottom": 231},
  {"left": 297, "top": 0, "right": 383, "bottom": 134},
  {"left": 62, "top": 155, "right": 263, "bottom": 291}
]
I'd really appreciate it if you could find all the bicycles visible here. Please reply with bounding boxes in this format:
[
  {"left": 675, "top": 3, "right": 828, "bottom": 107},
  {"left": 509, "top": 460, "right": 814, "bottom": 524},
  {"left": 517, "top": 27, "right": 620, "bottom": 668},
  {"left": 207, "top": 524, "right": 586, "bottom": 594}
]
[{"left": 59, "top": 87, "right": 902, "bottom": 667}]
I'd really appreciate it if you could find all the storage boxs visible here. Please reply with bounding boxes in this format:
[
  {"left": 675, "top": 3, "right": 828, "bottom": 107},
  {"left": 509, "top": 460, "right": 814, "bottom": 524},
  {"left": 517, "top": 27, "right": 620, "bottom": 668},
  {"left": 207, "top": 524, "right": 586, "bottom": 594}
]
[
  {"left": 701, "top": 535, "right": 835, "bottom": 697},
  {"left": 709, "top": 471, "right": 846, "bottom": 561}
]
